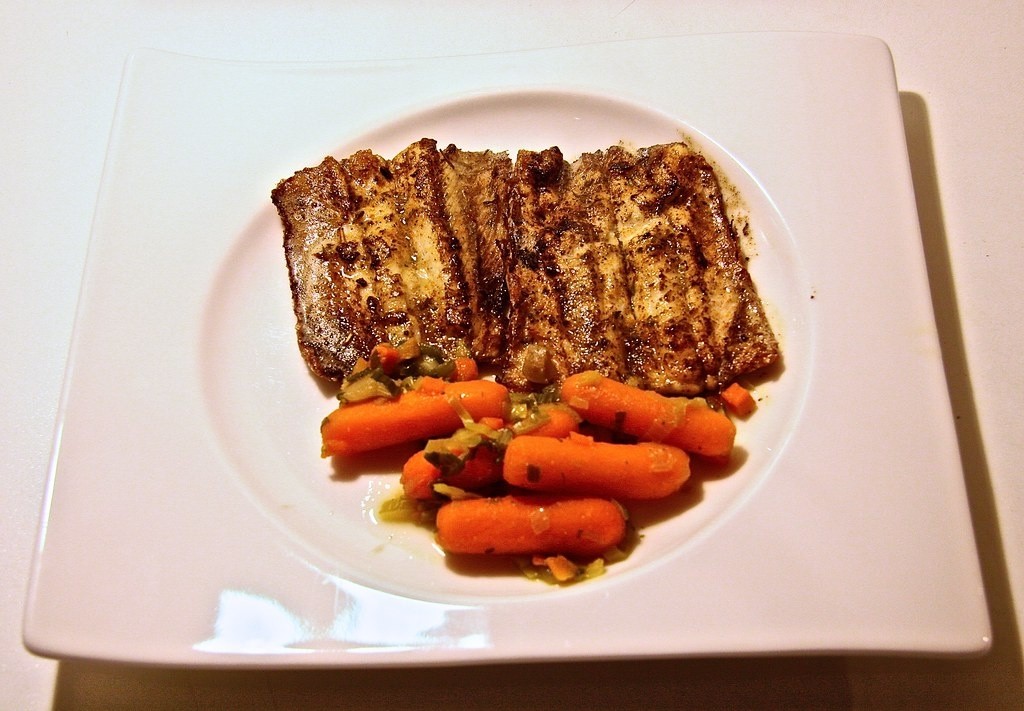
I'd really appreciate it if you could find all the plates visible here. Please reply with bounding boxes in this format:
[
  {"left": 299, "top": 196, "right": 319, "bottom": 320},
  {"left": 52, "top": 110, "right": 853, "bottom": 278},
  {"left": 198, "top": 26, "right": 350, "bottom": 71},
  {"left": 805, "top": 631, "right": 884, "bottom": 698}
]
[{"left": 20, "top": 32, "right": 992, "bottom": 672}]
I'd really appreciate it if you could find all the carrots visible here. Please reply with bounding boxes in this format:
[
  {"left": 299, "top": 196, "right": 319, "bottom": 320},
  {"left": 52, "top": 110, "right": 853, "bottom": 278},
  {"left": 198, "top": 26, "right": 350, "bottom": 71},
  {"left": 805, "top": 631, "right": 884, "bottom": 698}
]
[{"left": 321, "top": 372, "right": 757, "bottom": 558}]
